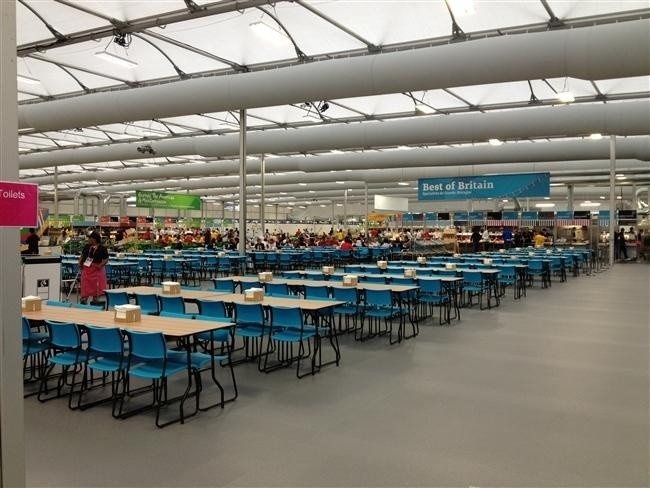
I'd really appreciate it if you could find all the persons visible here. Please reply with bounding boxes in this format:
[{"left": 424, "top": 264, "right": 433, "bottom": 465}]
[
  {"left": 42, "top": 224, "right": 650, "bottom": 265},
  {"left": 78, "top": 231, "right": 109, "bottom": 304},
  {"left": 20, "top": 228, "right": 40, "bottom": 254}
]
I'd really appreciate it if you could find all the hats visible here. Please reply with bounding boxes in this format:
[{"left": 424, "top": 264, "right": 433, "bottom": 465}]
[{"left": 86, "top": 231, "right": 100, "bottom": 239}]
